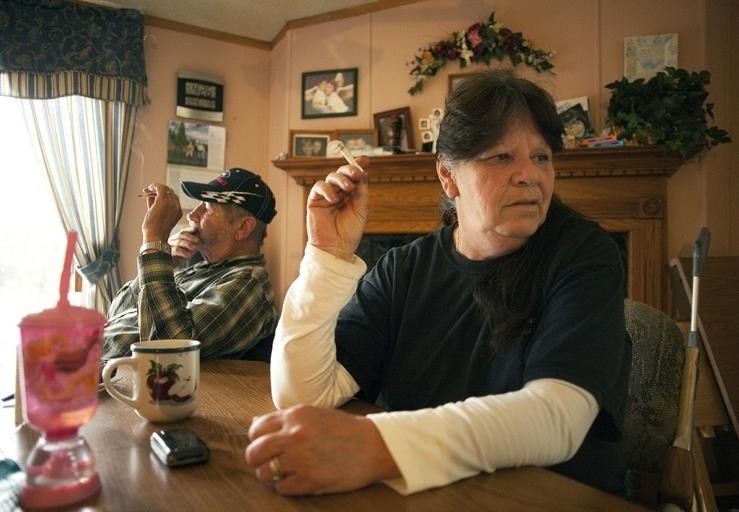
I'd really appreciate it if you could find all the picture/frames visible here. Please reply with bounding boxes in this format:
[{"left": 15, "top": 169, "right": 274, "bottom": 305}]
[
  {"left": 288, "top": 128, "right": 376, "bottom": 160},
  {"left": 301, "top": 67, "right": 357, "bottom": 117},
  {"left": 448, "top": 72, "right": 474, "bottom": 92},
  {"left": 372, "top": 105, "right": 416, "bottom": 152},
  {"left": 623, "top": 32, "right": 679, "bottom": 82}
]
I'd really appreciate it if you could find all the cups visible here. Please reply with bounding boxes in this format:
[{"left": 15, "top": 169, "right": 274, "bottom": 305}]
[{"left": 101, "top": 338, "right": 202, "bottom": 428}]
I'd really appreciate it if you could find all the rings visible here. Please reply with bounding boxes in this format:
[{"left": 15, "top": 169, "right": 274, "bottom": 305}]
[
  {"left": 267, "top": 458, "right": 286, "bottom": 481},
  {"left": 166, "top": 190, "right": 172, "bottom": 195}
]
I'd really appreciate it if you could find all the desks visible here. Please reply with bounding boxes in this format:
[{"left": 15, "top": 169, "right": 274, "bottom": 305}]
[{"left": 0, "top": 356, "right": 654, "bottom": 512}]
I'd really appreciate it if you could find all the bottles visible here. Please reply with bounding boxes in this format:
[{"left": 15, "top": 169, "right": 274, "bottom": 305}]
[{"left": 17, "top": 301, "right": 107, "bottom": 512}]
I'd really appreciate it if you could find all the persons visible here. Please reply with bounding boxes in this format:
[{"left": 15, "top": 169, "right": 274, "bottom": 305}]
[
  {"left": 312, "top": 138, "right": 324, "bottom": 156},
  {"left": 246, "top": 72, "right": 633, "bottom": 499},
  {"left": 100, "top": 168, "right": 278, "bottom": 362},
  {"left": 312, "top": 73, "right": 352, "bottom": 112},
  {"left": 561, "top": 109, "right": 587, "bottom": 139},
  {"left": 300, "top": 138, "right": 313, "bottom": 156}
]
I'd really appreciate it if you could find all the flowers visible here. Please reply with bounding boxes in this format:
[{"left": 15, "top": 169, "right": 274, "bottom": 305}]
[{"left": 391, "top": 11, "right": 558, "bottom": 97}]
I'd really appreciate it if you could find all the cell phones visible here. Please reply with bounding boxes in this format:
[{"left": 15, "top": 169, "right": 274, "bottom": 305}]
[{"left": 148, "top": 427, "right": 209, "bottom": 468}]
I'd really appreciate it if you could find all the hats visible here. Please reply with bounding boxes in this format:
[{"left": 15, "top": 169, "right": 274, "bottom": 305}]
[{"left": 181, "top": 169, "right": 276, "bottom": 224}]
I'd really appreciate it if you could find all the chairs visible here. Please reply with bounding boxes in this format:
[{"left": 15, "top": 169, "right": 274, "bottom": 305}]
[{"left": 593, "top": 298, "right": 688, "bottom": 505}]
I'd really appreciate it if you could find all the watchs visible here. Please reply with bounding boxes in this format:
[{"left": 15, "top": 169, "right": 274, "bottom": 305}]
[{"left": 137, "top": 241, "right": 172, "bottom": 255}]
